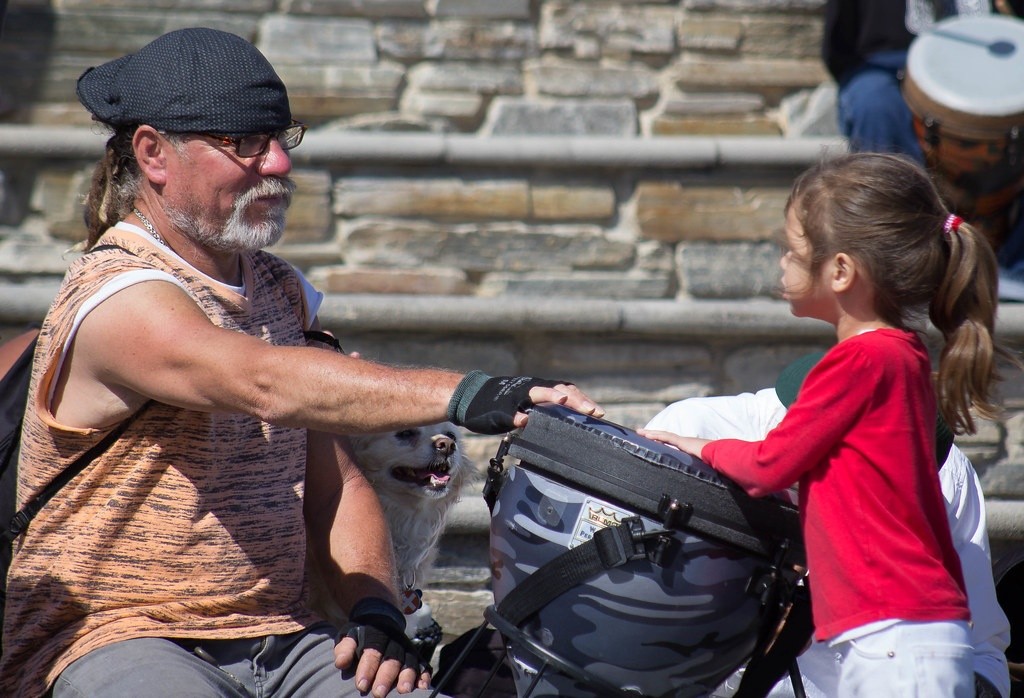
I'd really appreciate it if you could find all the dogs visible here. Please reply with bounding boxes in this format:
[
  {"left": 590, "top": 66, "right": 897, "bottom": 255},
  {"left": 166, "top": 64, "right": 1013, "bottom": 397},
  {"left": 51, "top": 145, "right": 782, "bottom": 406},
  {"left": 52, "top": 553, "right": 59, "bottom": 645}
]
[{"left": 349, "top": 419, "right": 477, "bottom": 640}]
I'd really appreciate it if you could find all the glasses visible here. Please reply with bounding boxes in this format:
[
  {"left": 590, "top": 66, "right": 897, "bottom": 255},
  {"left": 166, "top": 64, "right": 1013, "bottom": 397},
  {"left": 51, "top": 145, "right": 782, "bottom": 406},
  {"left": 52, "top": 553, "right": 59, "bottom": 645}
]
[{"left": 196, "top": 119, "right": 309, "bottom": 158}]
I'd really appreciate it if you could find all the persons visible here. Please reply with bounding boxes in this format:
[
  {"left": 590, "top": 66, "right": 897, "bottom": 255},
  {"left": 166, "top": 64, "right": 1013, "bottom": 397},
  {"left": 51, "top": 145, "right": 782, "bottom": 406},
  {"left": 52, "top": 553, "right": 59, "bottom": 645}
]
[
  {"left": 642, "top": 356, "right": 1013, "bottom": 698},
  {"left": 0, "top": 27, "right": 604, "bottom": 698},
  {"left": 636, "top": 153, "right": 998, "bottom": 698},
  {"left": 822, "top": 0, "right": 1024, "bottom": 302}
]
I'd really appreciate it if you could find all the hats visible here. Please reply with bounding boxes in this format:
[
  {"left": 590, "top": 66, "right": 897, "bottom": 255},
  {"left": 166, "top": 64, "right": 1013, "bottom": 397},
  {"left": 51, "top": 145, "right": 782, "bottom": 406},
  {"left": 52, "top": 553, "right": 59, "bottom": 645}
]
[
  {"left": 76, "top": 28, "right": 292, "bottom": 131},
  {"left": 775, "top": 349, "right": 955, "bottom": 473}
]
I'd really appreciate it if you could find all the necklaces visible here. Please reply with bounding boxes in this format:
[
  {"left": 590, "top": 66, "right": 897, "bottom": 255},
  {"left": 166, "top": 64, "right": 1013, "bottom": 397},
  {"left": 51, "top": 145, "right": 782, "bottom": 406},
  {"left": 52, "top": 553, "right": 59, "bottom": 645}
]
[{"left": 131, "top": 207, "right": 166, "bottom": 246}]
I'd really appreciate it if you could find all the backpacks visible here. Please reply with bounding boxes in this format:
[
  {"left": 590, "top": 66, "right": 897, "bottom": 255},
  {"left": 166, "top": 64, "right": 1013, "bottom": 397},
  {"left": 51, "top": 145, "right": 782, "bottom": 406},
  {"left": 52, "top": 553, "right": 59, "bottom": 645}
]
[{"left": 0, "top": 244, "right": 154, "bottom": 657}]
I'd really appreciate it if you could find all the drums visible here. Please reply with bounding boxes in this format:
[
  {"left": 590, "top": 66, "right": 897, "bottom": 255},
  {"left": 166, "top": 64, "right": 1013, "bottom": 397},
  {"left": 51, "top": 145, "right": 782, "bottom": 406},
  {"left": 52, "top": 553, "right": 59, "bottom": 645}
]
[
  {"left": 485, "top": 403, "right": 811, "bottom": 696},
  {"left": 898, "top": 11, "right": 1024, "bottom": 267}
]
